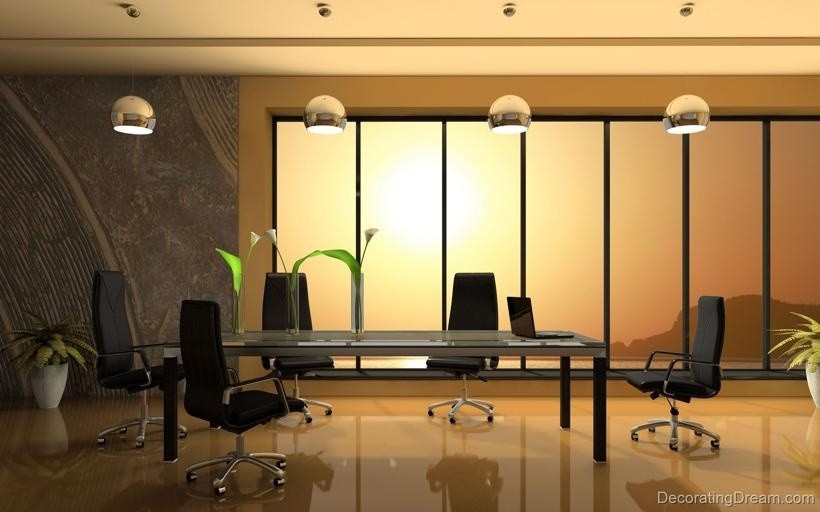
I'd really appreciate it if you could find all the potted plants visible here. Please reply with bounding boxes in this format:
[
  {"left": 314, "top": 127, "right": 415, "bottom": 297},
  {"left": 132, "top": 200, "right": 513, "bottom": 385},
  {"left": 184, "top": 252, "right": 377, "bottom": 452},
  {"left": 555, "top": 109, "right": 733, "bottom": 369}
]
[
  {"left": 3, "top": 309, "right": 97, "bottom": 409},
  {"left": 767, "top": 310, "right": 819, "bottom": 410}
]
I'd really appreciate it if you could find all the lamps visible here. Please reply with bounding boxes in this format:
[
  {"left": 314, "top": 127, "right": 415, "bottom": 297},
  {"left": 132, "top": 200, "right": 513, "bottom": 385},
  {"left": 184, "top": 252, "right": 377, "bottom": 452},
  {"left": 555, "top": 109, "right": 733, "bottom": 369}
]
[
  {"left": 488, "top": 2, "right": 531, "bottom": 136},
  {"left": 303, "top": 1, "right": 347, "bottom": 136},
  {"left": 109, "top": 0, "right": 156, "bottom": 136},
  {"left": 663, "top": 4, "right": 710, "bottom": 136}
]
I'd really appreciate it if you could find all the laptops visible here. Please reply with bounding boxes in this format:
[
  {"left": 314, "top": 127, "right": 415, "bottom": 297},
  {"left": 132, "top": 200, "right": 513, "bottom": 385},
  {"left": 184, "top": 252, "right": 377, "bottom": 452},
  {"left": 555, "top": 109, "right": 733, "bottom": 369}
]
[{"left": 507, "top": 297, "right": 573, "bottom": 339}]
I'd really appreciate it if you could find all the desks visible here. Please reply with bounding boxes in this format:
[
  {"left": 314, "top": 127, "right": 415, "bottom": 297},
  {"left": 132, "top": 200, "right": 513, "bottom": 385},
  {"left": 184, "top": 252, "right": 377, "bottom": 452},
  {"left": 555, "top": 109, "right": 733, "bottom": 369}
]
[{"left": 152, "top": 328, "right": 609, "bottom": 465}]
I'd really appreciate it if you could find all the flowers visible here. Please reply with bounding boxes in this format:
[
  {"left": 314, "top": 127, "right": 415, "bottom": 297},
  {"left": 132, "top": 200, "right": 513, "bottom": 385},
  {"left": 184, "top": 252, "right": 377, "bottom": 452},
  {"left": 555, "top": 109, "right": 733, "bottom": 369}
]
[{"left": 214, "top": 228, "right": 379, "bottom": 335}]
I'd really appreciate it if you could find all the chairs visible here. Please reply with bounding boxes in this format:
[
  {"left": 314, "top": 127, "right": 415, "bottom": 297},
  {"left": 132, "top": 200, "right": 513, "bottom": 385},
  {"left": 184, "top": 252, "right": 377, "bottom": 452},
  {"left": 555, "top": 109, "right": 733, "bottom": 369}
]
[
  {"left": 625, "top": 295, "right": 727, "bottom": 452},
  {"left": 425, "top": 271, "right": 500, "bottom": 426},
  {"left": 87, "top": 273, "right": 333, "bottom": 495}
]
